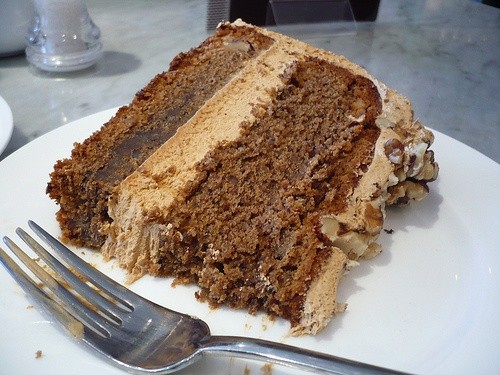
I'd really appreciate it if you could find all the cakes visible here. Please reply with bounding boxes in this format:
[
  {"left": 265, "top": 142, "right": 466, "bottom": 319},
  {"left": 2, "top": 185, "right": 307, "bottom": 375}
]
[{"left": 45, "top": 19, "right": 440, "bottom": 337}]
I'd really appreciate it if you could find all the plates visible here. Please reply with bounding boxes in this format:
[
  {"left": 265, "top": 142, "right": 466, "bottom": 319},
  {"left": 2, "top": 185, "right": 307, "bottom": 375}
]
[{"left": 0, "top": 99, "right": 500, "bottom": 375}]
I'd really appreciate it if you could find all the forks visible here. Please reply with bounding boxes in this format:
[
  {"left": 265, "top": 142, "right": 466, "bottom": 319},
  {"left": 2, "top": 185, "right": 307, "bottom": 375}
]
[{"left": 0, "top": 220, "right": 415, "bottom": 375}]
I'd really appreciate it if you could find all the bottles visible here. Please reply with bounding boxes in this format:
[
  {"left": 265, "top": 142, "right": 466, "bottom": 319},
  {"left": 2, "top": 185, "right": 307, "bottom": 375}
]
[{"left": 23, "top": 1, "right": 102, "bottom": 72}]
